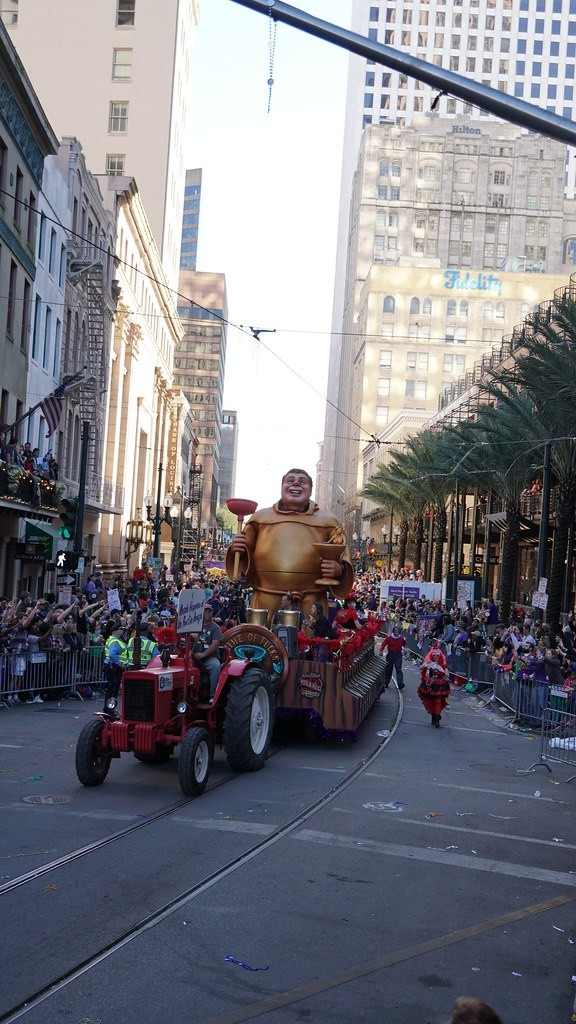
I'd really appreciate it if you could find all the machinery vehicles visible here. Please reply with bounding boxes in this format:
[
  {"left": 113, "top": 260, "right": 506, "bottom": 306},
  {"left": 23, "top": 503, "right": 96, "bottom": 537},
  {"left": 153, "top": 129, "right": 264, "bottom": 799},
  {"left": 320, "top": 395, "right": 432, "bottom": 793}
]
[{"left": 75, "top": 608, "right": 277, "bottom": 798}]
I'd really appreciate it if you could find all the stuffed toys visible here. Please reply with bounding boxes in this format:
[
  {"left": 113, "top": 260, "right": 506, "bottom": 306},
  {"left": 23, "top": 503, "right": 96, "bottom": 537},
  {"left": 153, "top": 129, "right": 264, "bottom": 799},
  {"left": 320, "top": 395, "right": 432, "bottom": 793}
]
[{"left": 332, "top": 597, "right": 363, "bottom": 631}]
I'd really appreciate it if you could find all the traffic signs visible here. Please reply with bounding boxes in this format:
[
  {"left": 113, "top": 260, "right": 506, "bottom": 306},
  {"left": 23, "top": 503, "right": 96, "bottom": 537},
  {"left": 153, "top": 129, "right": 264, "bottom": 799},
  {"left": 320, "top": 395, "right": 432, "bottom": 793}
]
[{"left": 56, "top": 574, "right": 78, "bottom": 586}]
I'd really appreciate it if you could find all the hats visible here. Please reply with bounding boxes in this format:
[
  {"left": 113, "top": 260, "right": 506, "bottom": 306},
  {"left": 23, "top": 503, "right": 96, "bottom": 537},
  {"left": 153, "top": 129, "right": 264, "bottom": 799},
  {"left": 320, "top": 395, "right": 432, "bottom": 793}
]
[
  {"left": 141, "top": 623, "right": 148, "bottom": 631},
  {"left": 111, "top": 609, "right": 125, "bottom": 616},
  {"left": 204, "top": 604, "right": 213, "bottom": 610},
  {"left": 496, "top": 624, "right": 504, "bottom": 628},
  {"left": 111, "top": 622, "right": 126, "bottom": 631}
]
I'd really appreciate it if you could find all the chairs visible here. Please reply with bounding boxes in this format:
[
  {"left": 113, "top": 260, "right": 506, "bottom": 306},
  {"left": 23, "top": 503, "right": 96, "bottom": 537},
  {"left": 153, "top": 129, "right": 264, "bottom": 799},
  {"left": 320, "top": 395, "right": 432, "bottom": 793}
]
[{"left": 181, "top": 640, "right": 231, "bottom": 706}]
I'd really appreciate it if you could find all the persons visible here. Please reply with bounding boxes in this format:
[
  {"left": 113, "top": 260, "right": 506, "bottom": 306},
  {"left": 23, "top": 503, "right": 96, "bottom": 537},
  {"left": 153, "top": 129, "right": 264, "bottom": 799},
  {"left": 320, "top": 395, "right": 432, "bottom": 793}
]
[
  {"left": 104, "top": 622, "right": 129, "bottom": 721},
  {"left": 349, "top": 565, "right": 576, "bottom": 727},
  {"left": 0, "top": 557, "right": 258, "bottom": 707},
  {"left": 309, "top": 604, "right": 339, "bottom": 639},
  {"left": 272, "top": 595, "right": 306, "bottom": 634},
  {"left": 225, "top": 469, "right": 354, "bottom": 639},
  {"left": 0, "top": 431, "right": 61, "bottom": 481}
]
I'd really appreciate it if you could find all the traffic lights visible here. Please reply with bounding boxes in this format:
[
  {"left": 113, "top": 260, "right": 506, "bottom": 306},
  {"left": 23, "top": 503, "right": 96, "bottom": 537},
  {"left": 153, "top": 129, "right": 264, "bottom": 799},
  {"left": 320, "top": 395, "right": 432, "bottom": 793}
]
[
  {"left": 59, "top": 498, "right": 78, "bottom": 541},
  {"left": 56, "top": 550, "right": 79, "bottom": 570}
]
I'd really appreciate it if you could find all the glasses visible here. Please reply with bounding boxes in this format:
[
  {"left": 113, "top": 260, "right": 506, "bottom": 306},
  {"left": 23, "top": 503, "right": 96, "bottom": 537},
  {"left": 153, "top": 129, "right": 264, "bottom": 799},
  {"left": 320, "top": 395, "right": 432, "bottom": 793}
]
[{"left": 118, "top": 613, "right": 122, "bottom": 614}]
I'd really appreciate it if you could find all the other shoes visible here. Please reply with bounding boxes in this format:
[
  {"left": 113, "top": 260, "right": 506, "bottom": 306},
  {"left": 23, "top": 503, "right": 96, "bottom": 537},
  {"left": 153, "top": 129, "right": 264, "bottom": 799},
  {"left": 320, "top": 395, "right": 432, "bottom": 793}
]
[
  {"left": 103, "top": 716, "right": 120, "bottom": 720},
  {"left": 499, "top": 706, "right": 510, "bottom": 712},
  {"left": 398, "top": 683, "right": 405, "bottom": 689},
  {"left": 209, "top": 698, "right": 214, "bottom": 705},
  {"left": 431, "top": 713, "right": 441, "bottom": 727},
  {"left": 14, "top": 699, "right": 21, "bottom": 702},
  {"left": 384, "top": 684, "right": 388, "bottom": 688},
  {"left": 33, "top": 696, "right": 44, "bottom": 702},
  {"left": 10, "top": 699, "right": 15, "bottom": 703}
]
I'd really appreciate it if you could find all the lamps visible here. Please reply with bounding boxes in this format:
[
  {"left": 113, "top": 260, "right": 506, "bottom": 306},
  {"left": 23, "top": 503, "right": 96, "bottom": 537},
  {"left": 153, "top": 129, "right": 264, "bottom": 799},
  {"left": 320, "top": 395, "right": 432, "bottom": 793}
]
[
  {"left": 125, "top": 507, "right": 145, "bottom": 558},
  {"left": 142, "top": 512, "right": 158, "bottom": 561}
]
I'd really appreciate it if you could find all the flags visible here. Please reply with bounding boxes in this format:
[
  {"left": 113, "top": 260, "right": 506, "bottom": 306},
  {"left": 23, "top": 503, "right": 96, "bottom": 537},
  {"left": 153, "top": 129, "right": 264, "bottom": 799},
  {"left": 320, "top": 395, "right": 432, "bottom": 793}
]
[{"left": 40, "top": 384, "right": 66, "bottom": 438}]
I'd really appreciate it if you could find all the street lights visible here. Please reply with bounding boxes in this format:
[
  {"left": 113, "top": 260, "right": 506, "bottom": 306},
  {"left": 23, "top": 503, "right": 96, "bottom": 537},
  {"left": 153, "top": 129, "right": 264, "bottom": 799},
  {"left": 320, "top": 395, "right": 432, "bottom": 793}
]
[
  {"left": 352, "top": 533, "right": 366, "bottom": 574},
  {"left": 192, "top": 520, "right": 207, "bottom": 571},
  {"left": 382, "top": 523, "right": 400, "bottom": 574},
  {"left": 143, "top": 490, "right": 173, "bottom": 589},
  {"left": 170, "top": 505, "right": 192, "bottom": 586}
]
